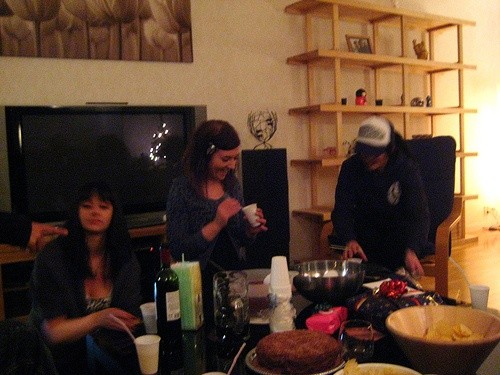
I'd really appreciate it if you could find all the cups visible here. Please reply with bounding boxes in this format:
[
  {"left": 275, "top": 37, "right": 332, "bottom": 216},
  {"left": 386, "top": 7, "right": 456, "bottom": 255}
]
[
  {"left": 140, "top": 302, "right": 157, "bottom": 333},
  {"left": 339, "top": 320, "right": 373, "bottom": 364},
  {"left": 212, "top": 271, "right": 249, "bottom": 341},
  {"left": 241, "top": 202, "right": 261, "bottom": 227},
  {"left": 134, "top": 335, "right": 161, "bottom": 374},
  {"left": 470, "top": 285, "right": 489, "bottom": 310}
]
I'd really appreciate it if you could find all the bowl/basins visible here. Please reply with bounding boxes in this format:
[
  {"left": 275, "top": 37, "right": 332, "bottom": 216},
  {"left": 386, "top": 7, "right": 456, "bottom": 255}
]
[
  {"left": 340, "top": 327, "right": 384, "bottom": 364},
  {"left": 240, "top": 269, "right": 298, "bottom": 324},
  {"left": 386, "top": 306, "right": 500, "bottom": 375},
  {"left": 293, "top": 259, "right": 364, "bottom": 302}
]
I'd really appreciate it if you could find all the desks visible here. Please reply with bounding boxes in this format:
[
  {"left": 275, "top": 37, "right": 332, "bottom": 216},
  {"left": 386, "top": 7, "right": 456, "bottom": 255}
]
[{"left": 0, "top": 224, "right": 168, "bottom": 322}]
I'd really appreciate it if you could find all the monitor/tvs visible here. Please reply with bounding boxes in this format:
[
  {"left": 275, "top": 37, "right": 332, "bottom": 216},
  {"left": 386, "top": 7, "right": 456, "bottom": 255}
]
[{"left": 5, "top": 104, "right": 196, "bottom": 234}]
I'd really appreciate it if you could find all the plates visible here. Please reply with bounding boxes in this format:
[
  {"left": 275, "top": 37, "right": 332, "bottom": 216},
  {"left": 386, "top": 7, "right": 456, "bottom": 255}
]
[
  {"left": 245, "top": 346, "right": 346, "bottom": 375},
  {"left": 334, "top": 362, "right": 423, "bottom": 375}
]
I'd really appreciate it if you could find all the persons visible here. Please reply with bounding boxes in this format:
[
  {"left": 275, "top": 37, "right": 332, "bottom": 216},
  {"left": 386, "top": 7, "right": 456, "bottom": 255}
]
[
  {"left": 166, "top": 119, "right": 270, "bottom": 278},
  {"left": 331, "top": 117, "right": 431, "bottom": 277},
  {"left": 0, "top": 183, "right": 143, "bottom": 375}
]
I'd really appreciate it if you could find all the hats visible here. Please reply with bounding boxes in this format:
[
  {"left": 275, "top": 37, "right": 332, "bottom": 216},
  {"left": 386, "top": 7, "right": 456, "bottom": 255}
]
[{"left": 356, "top": 115, "right": 392, "bottom": 148}]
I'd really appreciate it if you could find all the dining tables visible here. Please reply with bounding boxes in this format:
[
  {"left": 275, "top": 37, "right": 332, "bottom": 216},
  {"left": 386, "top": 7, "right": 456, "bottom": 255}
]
[{"left": 94, "top": 262, "right": 500, "bottom": 375}]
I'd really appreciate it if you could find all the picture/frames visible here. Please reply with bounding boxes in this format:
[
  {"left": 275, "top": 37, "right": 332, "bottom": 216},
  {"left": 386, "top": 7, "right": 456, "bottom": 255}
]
[{"left": 346, "top": 35, "right": 373, "bottom": 54}]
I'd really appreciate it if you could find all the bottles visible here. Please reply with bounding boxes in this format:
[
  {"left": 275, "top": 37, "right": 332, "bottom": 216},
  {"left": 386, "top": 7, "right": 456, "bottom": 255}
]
[
  {"left": 153, "top": 243, "right": 182, "bottom": 345},
  {"left": 269, "top": 255, "right": 295, "bottom": 333}
]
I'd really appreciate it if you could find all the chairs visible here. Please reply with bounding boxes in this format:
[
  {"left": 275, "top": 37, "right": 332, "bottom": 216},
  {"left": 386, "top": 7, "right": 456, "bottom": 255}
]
[{"left": 321, "top": 136, "right": 467, "bottom": 297}]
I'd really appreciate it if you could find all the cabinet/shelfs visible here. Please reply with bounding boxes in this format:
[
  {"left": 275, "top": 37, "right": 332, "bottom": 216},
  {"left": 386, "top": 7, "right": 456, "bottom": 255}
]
[{"left": 284, "top": 0, "right": 478, "bottom": 261}]
